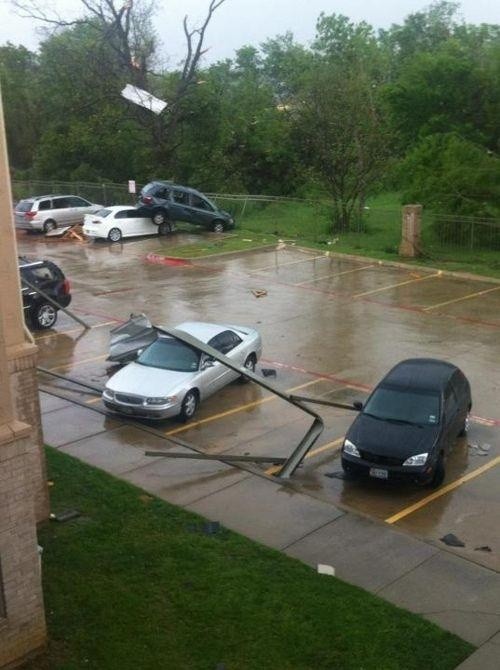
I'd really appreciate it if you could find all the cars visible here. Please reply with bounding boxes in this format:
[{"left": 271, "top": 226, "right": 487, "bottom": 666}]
[
  {"left": 340, "top": 357, "right": 473, "bottom": 485},
  {"left": 102, "top": 321, "right": 262, "bottom": 422},
  {"left": 20, "top": 253, "right": 71, "bottom": 330}
]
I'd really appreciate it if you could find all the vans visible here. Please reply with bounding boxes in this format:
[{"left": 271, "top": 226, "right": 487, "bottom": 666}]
[{"left": 14, "top": 180, "right": 234, "bottom": 242}]
